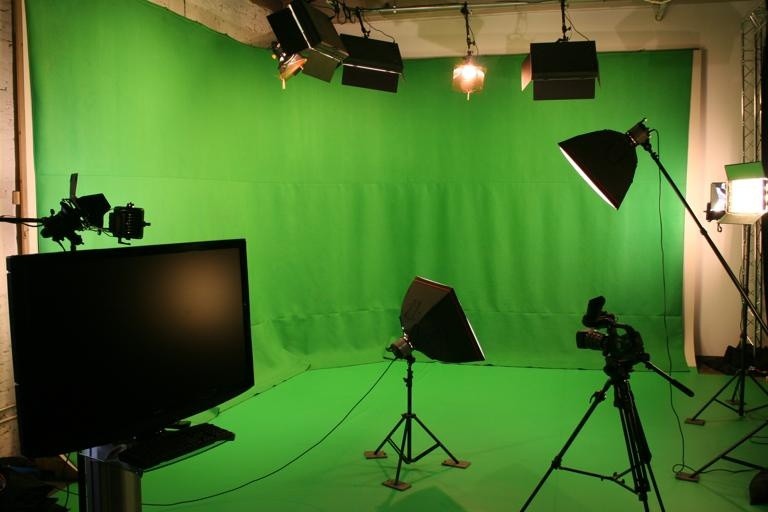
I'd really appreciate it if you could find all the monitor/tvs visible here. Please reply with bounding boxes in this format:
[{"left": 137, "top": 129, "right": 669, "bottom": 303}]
[{"left": 6, "top": 238, "right": 255, "bottom": 470}]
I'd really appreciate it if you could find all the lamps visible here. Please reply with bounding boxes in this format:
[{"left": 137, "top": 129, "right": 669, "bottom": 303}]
[
  {"left": 371, "top": 274, "right": 489, "bottom": 489},
  {"left": 686, "top": 162, "right": 768, "bottom": 424},
  {"left": 451, "top": 3, "right": 487, "bottom": 99},
  {"left": 552, "top": 114, "right": 767, "bottom": 340}
]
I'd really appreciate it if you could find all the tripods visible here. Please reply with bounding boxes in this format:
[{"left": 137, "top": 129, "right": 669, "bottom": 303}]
[
  {"left": 519, "top": 379, "right": 666, "bottom": 512},
  {"left": 374, "top": 359, "right": 459, "bottom": 485},
  {"left": 684, "top": 224, "right": 768, "bottom": 426}
]
